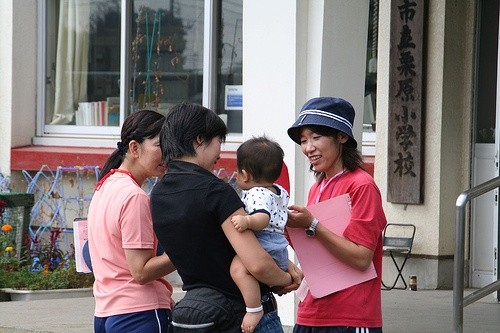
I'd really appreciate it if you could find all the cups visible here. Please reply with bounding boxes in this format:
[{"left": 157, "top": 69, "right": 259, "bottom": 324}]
[{"left": 409, "top": 275, "right": 417, "bottom": 291}]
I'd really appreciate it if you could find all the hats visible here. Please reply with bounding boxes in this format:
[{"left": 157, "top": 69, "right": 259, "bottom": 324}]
[{"left": 287, "top": 97, "right": 357, "bottom": 149}]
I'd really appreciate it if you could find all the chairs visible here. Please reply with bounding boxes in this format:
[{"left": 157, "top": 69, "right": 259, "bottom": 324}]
[{"left": 381, "top": 223, "right": 416, "bottom": 290}]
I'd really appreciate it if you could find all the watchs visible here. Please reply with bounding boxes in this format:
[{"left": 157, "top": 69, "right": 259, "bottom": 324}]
[{"left": 305, "top": 218, "right": 319, "bottom": 237}]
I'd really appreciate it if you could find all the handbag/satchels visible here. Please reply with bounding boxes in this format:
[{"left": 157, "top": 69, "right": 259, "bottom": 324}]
[{"left": 167, "top": 288, "right": 241, "bottom": 332}]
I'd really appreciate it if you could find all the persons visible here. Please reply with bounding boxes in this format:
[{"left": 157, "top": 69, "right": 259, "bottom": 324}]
[
  {"left": 228, "top": 133, "right": 289, "bottom": 332},
  {"left": 86, "top": 110, "right": 176, "bottom": 333},
  {"left": 284, "top": 96, "right": 387, "bottom": 333},
  {"left": 150, "top": 101, "right": 305, "bottom": 333}
]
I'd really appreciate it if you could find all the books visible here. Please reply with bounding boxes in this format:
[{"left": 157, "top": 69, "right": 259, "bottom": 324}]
[{"left": 76, "top": 95, "right": 178, "bottom": 126}]
